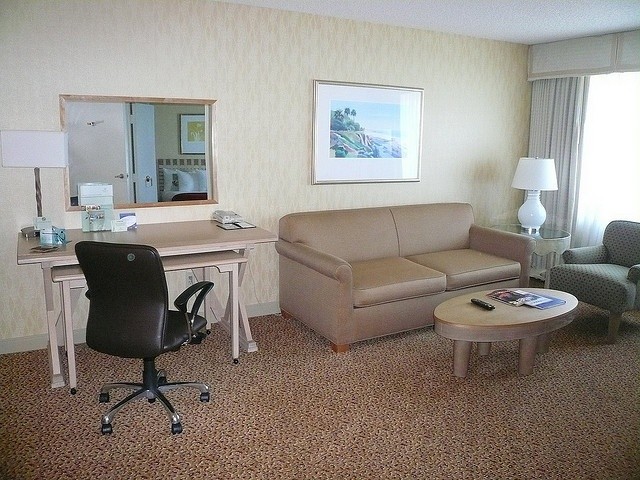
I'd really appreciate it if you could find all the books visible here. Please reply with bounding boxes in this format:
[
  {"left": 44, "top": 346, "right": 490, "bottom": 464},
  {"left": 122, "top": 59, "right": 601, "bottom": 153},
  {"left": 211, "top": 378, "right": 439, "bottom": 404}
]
[
  {"left": 525, "top": 293, "right": 566, "bottom": 310},
  {"left": 511, "top": 290, "right": 553, "bottom": 307},
  {"left": 487, "top": 289, "right": 532, "bottom": 307}
]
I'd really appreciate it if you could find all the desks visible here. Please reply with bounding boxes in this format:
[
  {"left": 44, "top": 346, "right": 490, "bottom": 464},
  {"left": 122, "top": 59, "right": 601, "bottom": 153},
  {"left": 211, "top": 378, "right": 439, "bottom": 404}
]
[
  {"left": 490, "top": 223, "right": 571, "bottom": 289},
  {"left": 18, "top": 220, "right": 278, "bottom": 388},
  {"left": 433, "top": 288, "right": 578, "bottom": 377}
]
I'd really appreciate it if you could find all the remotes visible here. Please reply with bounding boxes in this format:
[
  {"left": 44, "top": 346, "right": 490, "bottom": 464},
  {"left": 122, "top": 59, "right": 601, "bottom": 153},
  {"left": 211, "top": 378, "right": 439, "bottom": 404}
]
[{"left": 471, "top": 298, "right": 494, "bottom": 311}]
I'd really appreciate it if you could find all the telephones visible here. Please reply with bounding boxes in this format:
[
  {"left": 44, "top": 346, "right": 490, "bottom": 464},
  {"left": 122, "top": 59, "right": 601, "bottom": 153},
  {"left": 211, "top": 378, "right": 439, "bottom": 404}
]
[{"left": 212, "top": 210, "right": 243, "bottom": 224}]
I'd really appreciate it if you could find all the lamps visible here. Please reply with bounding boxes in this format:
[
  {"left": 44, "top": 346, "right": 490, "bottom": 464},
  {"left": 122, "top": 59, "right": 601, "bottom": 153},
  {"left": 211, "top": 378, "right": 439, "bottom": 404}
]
[
  {"left": 1, "top": 129, "right": 69, "bottom": 237},
  {"left": 510, "top": 157, "right": 558, "bottom": 232}
]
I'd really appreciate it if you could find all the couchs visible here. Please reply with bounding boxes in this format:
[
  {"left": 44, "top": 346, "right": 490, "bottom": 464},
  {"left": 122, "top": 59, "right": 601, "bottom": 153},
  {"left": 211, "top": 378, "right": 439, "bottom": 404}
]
[
  {"left": 274, "top": 202, "right": 537, "bottom": 351},
  {"left": 549, "top": 220, "right": 639, "bottom": 344}
]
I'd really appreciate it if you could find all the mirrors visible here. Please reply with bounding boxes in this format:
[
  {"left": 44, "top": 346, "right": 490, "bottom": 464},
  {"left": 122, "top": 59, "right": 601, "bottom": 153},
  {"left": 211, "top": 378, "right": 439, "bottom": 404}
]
[{"left": 58, "top": 95, "right": 220, "bottom": 211}]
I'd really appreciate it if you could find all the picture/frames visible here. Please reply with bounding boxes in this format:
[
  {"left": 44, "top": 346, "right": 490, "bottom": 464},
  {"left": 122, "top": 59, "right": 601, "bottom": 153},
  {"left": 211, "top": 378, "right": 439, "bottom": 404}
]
[{"left": 311, "top": 79, "right": 424, "bottom": 184}]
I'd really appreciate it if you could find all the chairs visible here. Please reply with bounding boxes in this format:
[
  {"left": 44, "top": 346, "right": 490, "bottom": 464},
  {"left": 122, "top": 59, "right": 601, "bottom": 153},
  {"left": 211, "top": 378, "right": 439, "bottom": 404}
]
[{"left": 74, "top": 241, "right": 214, "bottom": 434}]
